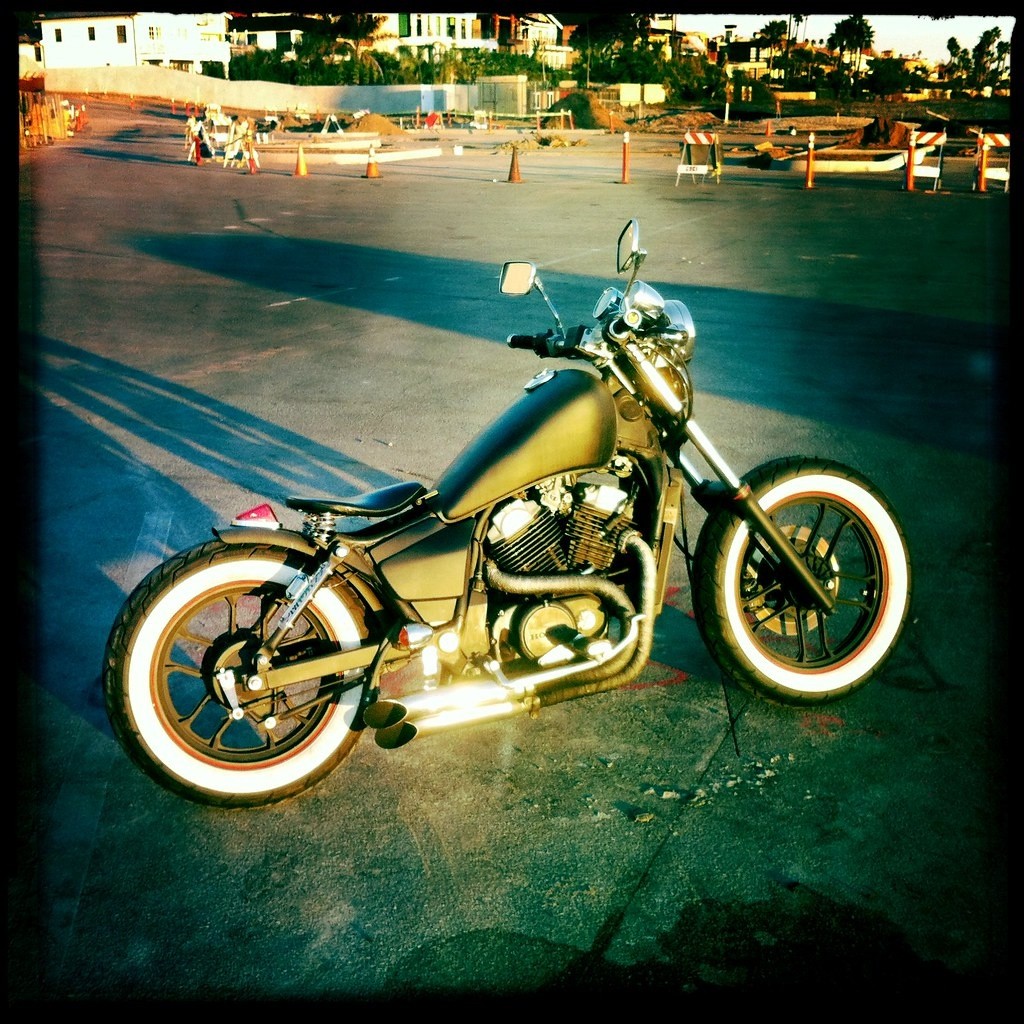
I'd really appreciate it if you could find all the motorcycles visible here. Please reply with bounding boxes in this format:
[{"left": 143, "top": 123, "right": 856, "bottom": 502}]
[{"left": 103, "top": 217, "right": 915, "bottom": 810}]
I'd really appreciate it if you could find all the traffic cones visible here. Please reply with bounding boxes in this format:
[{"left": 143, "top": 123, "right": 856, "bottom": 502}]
[
  {"left": 130, "top": 94, "right": 137, "bottom": 110},
  {"left": 502, "top": 145, "right": 529, "bottom": 184},
  {"left": 75, "top": 105, "right": 83, "bottom": 132},
  {"left": 360, "top": 143, "right": 384, "bottom": 179},
  {"left": 291, "top": 142, "right": 311, "bottom": 177},
  {"left": 765, "top": 121, "right": 773, "bottom": 137},
  {"left": 185, "top": 99, "right": 192, "bottom": 117},
  {"left": 81, "top": 104, "right": 91, "bottom": 125},
  {"left": 194, "top": 100, "right": 199, "bottom": 117},
  {"left": 170, "top": 97, "right": 177, "bottom": 115}
]
